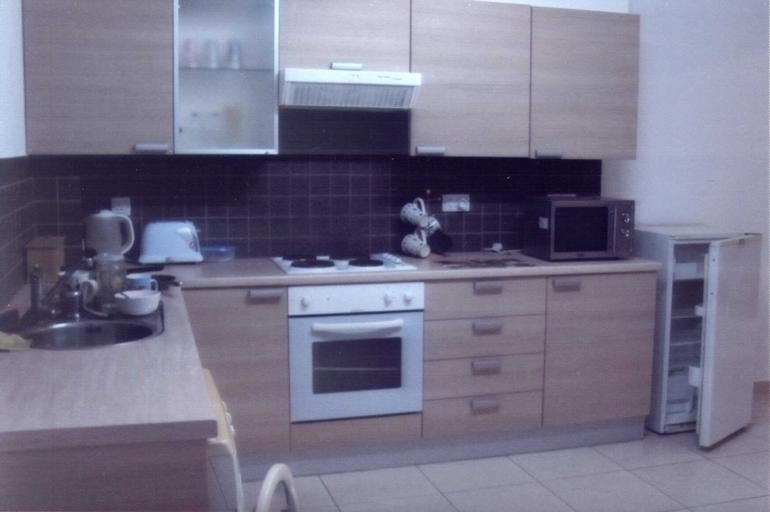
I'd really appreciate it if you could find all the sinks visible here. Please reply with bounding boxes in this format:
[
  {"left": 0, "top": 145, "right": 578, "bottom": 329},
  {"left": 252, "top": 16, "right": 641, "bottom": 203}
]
[{"left": 15, "top": 313, "right": 164, "bottom": 351}]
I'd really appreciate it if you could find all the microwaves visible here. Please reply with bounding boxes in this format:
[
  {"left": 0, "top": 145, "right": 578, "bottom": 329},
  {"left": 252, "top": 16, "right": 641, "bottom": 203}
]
[{"left": 525, "top": 189, "right": 636, "bottom": 264}]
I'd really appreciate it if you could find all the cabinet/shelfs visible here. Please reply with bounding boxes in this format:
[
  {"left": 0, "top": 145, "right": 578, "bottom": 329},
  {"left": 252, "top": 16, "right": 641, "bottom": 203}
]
[
  {"left": 167, "top": 255, "right": 292, "bottom": 482},
  {"left": 285, "top": 256, "right": 425, "bottom": 475},
  {"left": 530, "top": 254, "right": 663, "bottom": 453},
  {"left": 412, "top": 5, "right": 532, "bottom": 159},
  {"left": 1, "top": 257, "right": 243, "bottom": 510},
  {"left": 24, "top": 4, "right": 174, "bottom": 155},
  {"left": 400, "top": 256, "right": 548, "bottom": 465},
  {"left": 175, "top": 5, "right": 282, "bottom": 158},
  {"left": 274, "top": 3, "right": 413, "bottom": 158},
  {"left": 532, "top": 5, "right": 642, "bottom": 161}
]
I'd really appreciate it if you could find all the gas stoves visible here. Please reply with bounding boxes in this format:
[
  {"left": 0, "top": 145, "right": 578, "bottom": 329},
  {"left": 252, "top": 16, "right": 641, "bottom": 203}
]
[{"left": 271, "top": 250, "right": 419, "bottom": 275}]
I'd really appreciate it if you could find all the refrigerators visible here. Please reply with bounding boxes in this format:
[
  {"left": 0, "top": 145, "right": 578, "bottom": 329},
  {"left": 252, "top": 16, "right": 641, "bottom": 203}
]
[{"left": 636, "top": 224, "right": 764, "bottom": 449}]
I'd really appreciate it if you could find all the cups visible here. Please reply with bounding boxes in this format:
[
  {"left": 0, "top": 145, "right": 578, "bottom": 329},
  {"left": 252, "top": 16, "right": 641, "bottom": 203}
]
[
  {"left": 56, "top": 267, "right": 100, "bottom": 321},
  {"left": 400, "top": 229, "right": 431, "bottom": 258},
  {"left": 416, "top": 216, "right": 441, "bottom": 237},
  {"left": 399, "top": 197, "right": 428, "bottom": 228},
  {"left": 126, "top": 272, "right": 158, "bottom": 291}
]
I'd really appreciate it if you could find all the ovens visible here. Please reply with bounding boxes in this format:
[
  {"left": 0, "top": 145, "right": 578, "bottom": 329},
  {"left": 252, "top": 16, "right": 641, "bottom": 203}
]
[{"left": 285, "top": 280, "right": 429, "bottom": 427}]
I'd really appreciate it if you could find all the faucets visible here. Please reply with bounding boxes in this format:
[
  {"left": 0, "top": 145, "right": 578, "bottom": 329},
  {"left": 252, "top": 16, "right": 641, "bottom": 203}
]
[{"left": 29, "top": 257, "right": 94, "bottom": 317}]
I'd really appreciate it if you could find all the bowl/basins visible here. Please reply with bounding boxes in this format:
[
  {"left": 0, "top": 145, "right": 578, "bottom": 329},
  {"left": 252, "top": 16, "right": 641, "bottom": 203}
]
[{"left": 114, "top": 289, "right": 161, "bottom": 318}]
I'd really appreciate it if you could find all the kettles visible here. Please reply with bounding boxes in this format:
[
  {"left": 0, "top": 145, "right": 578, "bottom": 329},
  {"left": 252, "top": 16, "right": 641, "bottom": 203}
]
[{"left": 80, "top": 206, "right": 135, "bottom": 268}]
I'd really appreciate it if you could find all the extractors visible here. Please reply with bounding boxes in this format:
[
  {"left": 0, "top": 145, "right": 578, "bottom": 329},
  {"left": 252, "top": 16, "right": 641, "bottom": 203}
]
[{"left": 279, "top": 67, "right": 424, "bottom": 111}]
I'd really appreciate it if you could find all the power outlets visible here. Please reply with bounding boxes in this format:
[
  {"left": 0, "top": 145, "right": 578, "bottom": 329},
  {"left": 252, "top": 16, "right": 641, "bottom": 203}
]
[
  {"left": 442, "top": 194, "right": 470, "bottom": 213},
  {"left": 111, "top": 197, "right": 131, "bottom": 218}
]
